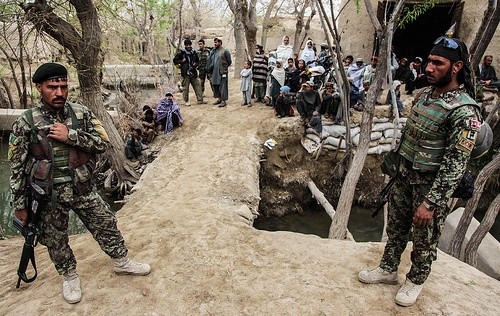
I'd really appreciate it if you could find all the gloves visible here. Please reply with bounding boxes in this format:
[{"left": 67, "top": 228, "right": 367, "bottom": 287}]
[{"left": 176, "top": 64, "right": 181, "bottom": 67}]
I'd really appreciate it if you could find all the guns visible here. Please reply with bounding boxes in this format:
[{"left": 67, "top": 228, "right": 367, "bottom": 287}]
[
  {"left": 372, "top": 178, "right": 396, "bottom": 218},
  {"left": 12, "top": 182, "right": 46, "bottom": 289}
]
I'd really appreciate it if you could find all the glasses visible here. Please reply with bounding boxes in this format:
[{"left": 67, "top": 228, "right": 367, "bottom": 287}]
[{"left": 434, "top": 36, "right": 459, "bottom": 52}]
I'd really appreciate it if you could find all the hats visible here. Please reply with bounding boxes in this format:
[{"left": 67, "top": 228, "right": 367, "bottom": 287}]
[
  {"left": 324, "top": 82, "right": 335, "bottom": 89},
  {"left": 32, "top": 63, "right": 67, "bottom": 83},
  {"left": 431, "top": 37, "right": 468, "bottom": 61},
  {"left": 184, "top": 40, "right": 192, "bottom": 45},
  {"left": 280, "top": 86, "right": 290, "bottom": 95},
  {"left": 357, "top": 58, "right": 364, "bottom": 62},
  {"left": 302, "top": 81, "right": 314, "bottom": 87},
  {"left": 321, "top": 43, "right": 328, "bottom": 48}
]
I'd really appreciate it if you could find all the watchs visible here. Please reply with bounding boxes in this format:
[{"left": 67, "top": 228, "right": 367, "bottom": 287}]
[
  {"left": 67, "top": 128, "right": 77, "bottom": 145},
  {"left": 423, "top": 201, "right": 435, "bottom": 212}
]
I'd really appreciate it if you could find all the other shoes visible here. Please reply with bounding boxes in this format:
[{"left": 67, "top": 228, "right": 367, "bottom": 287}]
[
  {"left": 214, "top": 100, "right": 221, "bottom": 104},
  {"left": 185, "top": 102, "right": 191, "bottom": 105},
  {"left": 198, "top": 101, "right": 206, "bottom": 104},
  {"left": 219, "top": 102, "right": 226, "bottom": 106}
]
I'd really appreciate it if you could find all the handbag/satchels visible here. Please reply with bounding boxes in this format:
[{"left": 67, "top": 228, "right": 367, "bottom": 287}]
[{"left": 289, "top": 106, "right": 294, "bottom": 115}]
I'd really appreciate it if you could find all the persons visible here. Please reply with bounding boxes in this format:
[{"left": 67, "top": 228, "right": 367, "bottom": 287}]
[
  {"left": 295, "top": 81, "right": 323, "bottom": 131},
  {"left": 266, "top": 35, "right": 428, "bottom": 119},
  {"left": 195, "top": 39, "right": 214, "bottom": 97},
  {"left": 139, "top": 105, "right": 157, "bottom": 131},
  {"left": 157, "top": 93, "right": 184, "bottom": 132},
  {"left": 475, "top": 55, "right": 498, "bottom": 86},
  {"left": 173, "top": 39, "right": 207, "bottom": 105},
  {"left": 358, "top": 37, "right": 482, "bottom": 306},
  {"left": 206, "top": 38, "right": 232, "bottom": 107},
  {"left": 7, "top": 63, "right": 151, "bottom": 304},
  {"left": 252, "top": 45, "right": 268, "bottom": 103},
  {"left": 125, "top": 128, "right": 143, "bottom": 162},
  {"left": 240, "top": 60, "right": 253, "bottom": 107}
]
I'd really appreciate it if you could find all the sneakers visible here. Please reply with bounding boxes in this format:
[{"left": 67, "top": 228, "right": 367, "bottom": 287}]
[
  {"left": 63, "top": 273, "right": 81, "bottom": 303},
  {"left": 395, "top": 277, "right": 424, "bottom": 306},
  {"left": 358, "top": 265, "right": 398, "bottom": 284},
  {"left": 114, "top": 258, "right": 150, "bottom": 275}
]
